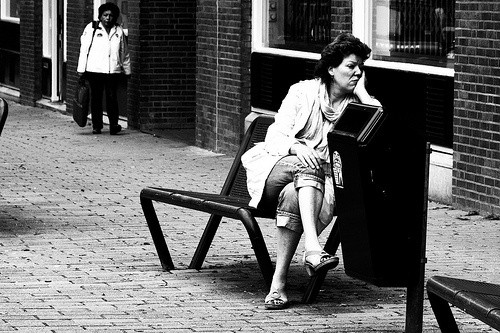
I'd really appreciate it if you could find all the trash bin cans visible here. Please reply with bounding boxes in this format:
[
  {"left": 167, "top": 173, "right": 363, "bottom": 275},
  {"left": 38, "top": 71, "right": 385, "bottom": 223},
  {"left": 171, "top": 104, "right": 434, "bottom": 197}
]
[{"left": 326, "top": 102, "right": 428, "bottom": 288}]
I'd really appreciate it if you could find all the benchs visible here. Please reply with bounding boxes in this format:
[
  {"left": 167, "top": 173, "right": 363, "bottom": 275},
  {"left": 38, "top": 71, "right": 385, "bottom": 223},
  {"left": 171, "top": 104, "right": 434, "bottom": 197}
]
[
  {"left": 426, "top": 276, "right": 500, "bottom": 333},
  {"left": 140, "top": 117, "right": 344, "bottom": 304}
]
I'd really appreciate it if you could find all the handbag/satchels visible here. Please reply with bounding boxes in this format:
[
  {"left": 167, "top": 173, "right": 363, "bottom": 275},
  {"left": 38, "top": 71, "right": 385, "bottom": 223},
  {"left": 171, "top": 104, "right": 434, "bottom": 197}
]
[{"left": 73, "top": 84, "right": 90, "bottom": 128}]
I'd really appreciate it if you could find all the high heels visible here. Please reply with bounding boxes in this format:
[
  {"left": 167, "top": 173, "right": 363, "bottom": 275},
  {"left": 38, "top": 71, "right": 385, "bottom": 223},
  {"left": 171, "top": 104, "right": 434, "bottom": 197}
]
[
  {"left": 302, "top": 250, "right": 340, "bottom": 277},
  {"left": 264, "top": 286, "right": 289, "bottom": 309}
]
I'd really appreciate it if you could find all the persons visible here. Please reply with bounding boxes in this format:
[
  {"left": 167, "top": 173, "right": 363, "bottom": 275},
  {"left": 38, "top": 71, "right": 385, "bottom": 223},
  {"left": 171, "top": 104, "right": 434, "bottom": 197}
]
[
  {"left": 239, "top": 34, "right": 386, "bottom": 307},
  {"left": 77, "top": 2, "right": 131, "bottom": 135}
]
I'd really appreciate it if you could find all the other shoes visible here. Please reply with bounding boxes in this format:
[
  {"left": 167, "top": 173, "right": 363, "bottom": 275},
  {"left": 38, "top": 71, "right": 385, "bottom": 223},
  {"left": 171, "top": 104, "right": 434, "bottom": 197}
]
[
  {"left": 110, "top": 124, "right": 122, "bottom": 135},
  {"left": 93, "top": 127, "right": 101, "bottom": 134}
]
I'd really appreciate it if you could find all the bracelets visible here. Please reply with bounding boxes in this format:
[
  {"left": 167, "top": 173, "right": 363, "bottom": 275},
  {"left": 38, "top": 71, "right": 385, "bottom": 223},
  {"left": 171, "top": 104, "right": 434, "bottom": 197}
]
[{"left": 289, "top": 148, "right": 291, "bottom": 155}]
